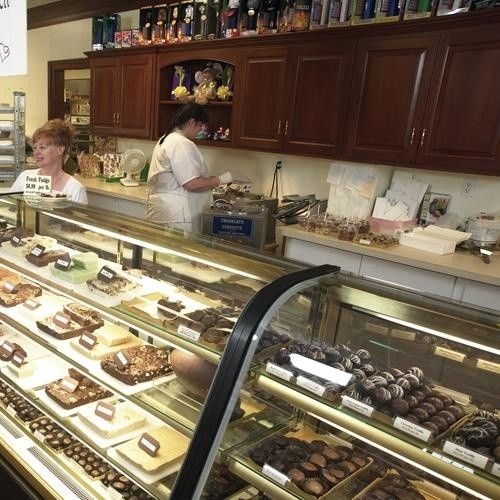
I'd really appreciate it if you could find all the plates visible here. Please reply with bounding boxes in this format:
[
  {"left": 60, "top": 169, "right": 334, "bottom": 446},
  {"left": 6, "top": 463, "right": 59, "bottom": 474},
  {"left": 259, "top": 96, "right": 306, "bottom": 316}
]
[{"left": 37, "top": 194, "right": 72, "bottom": 202}]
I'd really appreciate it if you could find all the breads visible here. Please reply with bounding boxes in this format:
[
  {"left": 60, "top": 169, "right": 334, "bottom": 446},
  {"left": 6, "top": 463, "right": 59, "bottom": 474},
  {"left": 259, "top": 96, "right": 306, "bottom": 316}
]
[
  {"left": 253, "top": 328, "right": 290, "bottom": 353},
  {"left": 249, "top": 434, "right": 368, "bottom": 496},
  {"left": 169, "top": 347, "right": 220, "bottom": 399},
  {"left": 199, "top": 464, "right": 251, "bottom": 500},
  {"left": 55, "top": 443, "right": 112, "bottom": 481},
  {"left": 77, "top": 150, "right": 99, "bottom": 177},
  {"left": 94, "top": 136, "right": 116, "bottom": 162},
  {"left": 450, "top": 401, "right": 500, "bottom": 463},
  {"left": 262, "top": 339, "right": 376, "bottom": 394},
  {"left": 354, "top": 366, "right": 467, "bottom": 438},
  {"left": 363, "top": 469, "right": 425, "bottom": 500},
  {"left": 103, "top": 153, "right": 124, "bottom": 179},
  {"left": 178, "top": 304, "right": 241, "bottom": 350},
  {"left": 0, "top": 378, "right": 42, "bottom": 422},
  {"left": 27, "top": 417, "right": 80, "bottom": 453},
  {"left": 91, "top": 471, "right": 159, "bottom": 500},
  {"left": 157, "top": 297, "right": 185, "bottom": 318}
]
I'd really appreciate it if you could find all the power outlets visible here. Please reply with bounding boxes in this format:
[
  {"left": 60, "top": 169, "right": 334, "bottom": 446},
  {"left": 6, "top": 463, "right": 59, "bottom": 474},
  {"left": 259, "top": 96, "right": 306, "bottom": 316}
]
[
  {"left": 462, "top": 179, "right": 474, "bottom": 196},
  {"left": 274, "top": 158, "right": 286, "bottom": 172}
]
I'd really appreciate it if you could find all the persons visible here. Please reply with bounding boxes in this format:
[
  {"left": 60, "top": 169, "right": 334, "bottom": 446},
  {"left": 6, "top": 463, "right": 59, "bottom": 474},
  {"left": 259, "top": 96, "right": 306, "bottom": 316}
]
[
  {"left": 8, "top": 122, "right": 88, "bottom": 205},
  {"left": 146, "top": 101, "right": 233, "bottom": 235}
]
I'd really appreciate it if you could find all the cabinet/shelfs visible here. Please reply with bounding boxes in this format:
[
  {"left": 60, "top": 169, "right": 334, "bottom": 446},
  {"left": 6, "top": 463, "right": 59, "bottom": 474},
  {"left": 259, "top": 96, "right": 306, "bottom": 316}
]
[
  {"left": 183, "top": 267, "right": 500, "bottom": 500},
  {"left": 343, "top": 9, "right": 500, "bottom": 177},
  {"left": 237, "top": 26, "right": 356, "bottom": 160},
  {"left": 0, "top": 92, "right": 26, "bottom": 187},
  {"left": 153, "top": 39, "right": 238, "bottom": 148},
  {"left": 70, "top": 101, "right": 90, "bottom": 155},
  {"left": 283, "top": 227, "right": 362, "bottom": 279},
  {"left": 0, "top": 193, "right": 328, "bottom": 500},
  {"left": 359, "top": 245, "right": 455, "bottom": 302},
  {"left": 84, "top": 47, "right": 154, "bottom": 139},
  {"left": 86, "top": 186, "right": 148, "bottom": 218},
  {"left": 451, "top": 276, "right": 500, "bottom": 312},
  {"left": 440, "top": 265, "right": 500, "bottom": 406}
]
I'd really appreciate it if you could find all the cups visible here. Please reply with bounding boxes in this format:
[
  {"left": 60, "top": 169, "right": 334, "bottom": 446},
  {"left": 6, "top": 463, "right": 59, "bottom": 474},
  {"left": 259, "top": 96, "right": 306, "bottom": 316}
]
[{"left": 298, "top": 214, "right": 370, "bottom": 241}]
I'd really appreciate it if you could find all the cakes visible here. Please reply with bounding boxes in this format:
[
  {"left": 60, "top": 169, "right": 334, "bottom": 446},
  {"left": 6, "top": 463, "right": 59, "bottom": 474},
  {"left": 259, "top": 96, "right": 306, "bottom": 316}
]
[
  {"left": 0, "top": 333, "right": 40, "bottom": 364},
  {"left": 86, "top": 266, "right": 136, "bottom": 297},
  {"left": 25, "top": 244, "right": 69, "bottom": 267},
  {"left": 352, "top": 232, "right": 399, "bottom": 249},
  {"left": 9, "top": 236, "right": 27, "bottom": 247},
  {"left": 69, "top": 324, "right": 142, "bottom": 359},
  {"left": 1, "top": 217, "right": 34, "bottom": 242},
  {"left": 78, "top": 404, "right": 146, "bottom": 440},
  {"left": 5, "top": 361, "right": 34, "bottom": 382},
  {"left": 45, "top": 366, "right": 114, "bottom": 410},
  {"left": 0, "top": 264, "right": 42, "bottom": 306},
  {"left": 53, "top": 256, "right": 75, "bottom": 271},
  {"left": 37, "top": 301, "right": 105, "bottom": 338},
  {"left": 117, "top": 425, "right": 192, "bottom": 471},
  {"left": 102, "top": 345, "right": 174, "bottom": 386},
  {"left": 299, "top": 212, "right": 370, "bottom": 241}
]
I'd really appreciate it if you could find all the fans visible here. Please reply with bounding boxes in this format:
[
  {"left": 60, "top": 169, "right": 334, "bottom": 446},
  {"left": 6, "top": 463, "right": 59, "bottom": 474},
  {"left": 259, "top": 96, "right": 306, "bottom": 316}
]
[{"left": 120, "top": 149, "right": 147, "bottom": 186}]
